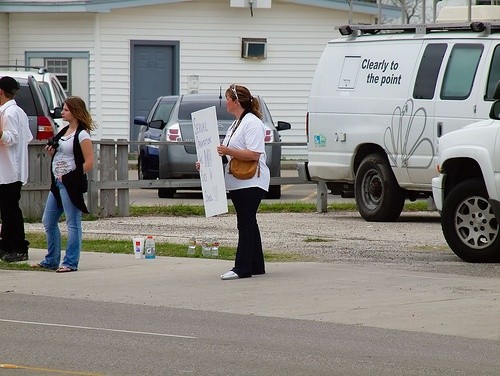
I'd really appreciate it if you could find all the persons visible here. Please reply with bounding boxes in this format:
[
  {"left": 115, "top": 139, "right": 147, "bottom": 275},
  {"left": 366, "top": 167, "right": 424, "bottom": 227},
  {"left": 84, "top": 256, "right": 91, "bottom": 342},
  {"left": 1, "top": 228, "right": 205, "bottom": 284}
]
[
  {"left": 0, "top": 76, "right": 34, "bottom": 261},
  {"left": 196, "top": 84, "right": 271, "bottom": 280},
  {"left": 31, "top": 95, "right": 99, "bottom": 273}
]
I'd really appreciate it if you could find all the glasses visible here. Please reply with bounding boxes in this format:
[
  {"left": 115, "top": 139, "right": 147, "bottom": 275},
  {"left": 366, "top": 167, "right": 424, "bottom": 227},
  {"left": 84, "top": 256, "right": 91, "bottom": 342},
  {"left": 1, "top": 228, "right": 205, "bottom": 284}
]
[{"left": 231, "top": 85, "right": 238, "bottom": 100}]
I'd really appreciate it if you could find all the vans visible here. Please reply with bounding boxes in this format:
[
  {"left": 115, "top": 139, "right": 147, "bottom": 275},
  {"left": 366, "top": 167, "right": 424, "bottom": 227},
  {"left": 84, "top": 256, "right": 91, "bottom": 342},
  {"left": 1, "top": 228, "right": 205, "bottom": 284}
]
[{"left": 295, "top": 18, "right": 500, "bottom": 222}]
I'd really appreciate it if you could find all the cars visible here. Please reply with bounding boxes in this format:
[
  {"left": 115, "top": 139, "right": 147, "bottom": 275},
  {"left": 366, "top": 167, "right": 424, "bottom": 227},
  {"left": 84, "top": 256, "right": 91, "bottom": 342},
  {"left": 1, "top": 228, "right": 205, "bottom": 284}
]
[
  {"left": 134, "top": 95, "right": 180, "bottom": 189},
  {"left": 147, "top": 92, "right": 292, "bottom": 200}
]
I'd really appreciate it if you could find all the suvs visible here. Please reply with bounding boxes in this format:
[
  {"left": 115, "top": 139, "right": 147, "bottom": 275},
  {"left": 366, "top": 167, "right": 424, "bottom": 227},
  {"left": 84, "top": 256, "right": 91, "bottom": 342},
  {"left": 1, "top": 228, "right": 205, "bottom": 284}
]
[
  {"left": 430, "top": 100, "right": 500, "bottom": 263},
  {"left": 0, "top": 74, "right": 64, "bottom": 143},
  {"left": 0, "top": 64, "right": 71, "bottom": 134}
]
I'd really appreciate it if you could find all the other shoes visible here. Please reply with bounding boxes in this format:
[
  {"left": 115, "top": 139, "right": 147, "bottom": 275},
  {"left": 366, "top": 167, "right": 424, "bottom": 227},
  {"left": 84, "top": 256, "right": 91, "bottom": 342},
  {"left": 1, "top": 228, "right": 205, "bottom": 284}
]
[
  {"left": 1, "top": 252, "right": 28, "bottom": 262},
  {"left": 56, "top": 266, "right": 72, "bottom": 272},
  {"left": 29, "top": 264, "right": 42, "bottom": 268},
  {"left": 220, "top": 271, "right": 238, "bottom": 279}
]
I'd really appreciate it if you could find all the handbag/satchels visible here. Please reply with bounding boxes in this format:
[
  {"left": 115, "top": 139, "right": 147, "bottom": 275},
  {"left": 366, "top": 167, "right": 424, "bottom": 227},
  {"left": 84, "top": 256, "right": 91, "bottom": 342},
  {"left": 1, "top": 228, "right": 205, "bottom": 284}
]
[{"left": 229, "top": 157, "right": 260, "bottom": 179}]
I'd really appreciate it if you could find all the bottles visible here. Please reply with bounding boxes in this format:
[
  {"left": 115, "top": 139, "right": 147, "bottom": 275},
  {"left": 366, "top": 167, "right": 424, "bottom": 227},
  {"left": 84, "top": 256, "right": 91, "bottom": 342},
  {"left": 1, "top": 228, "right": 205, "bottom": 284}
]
[
  {"left": 132, "top": 238, "right": 144, "bottom": 259},
  {"left": 187, "top": 235, "right": 220, "bottom": 257},
  {"left": 144, "top": 236, "right": 155, "bottom": 258}
]
